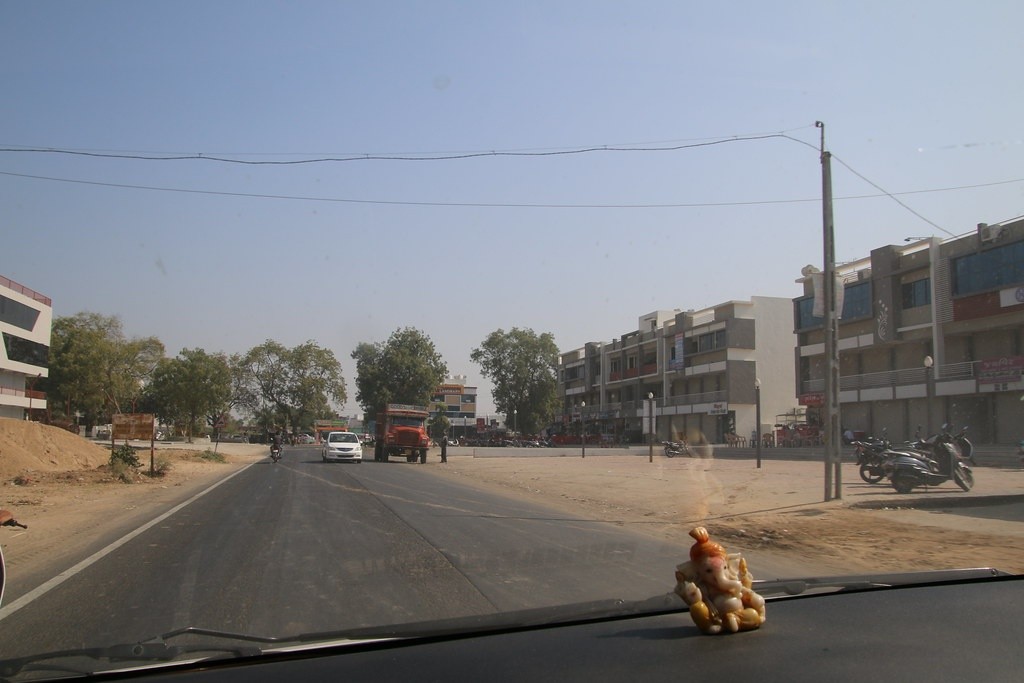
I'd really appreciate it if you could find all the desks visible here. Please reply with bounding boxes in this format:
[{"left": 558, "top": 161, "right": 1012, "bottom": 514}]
[{"left": 807, "top": 435, "right": 821, "bottom": 440}]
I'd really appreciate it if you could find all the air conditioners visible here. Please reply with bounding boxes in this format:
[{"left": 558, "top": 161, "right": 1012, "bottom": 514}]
[{"left": 980, "top": 224, "right": 1000, "bottom": 242}]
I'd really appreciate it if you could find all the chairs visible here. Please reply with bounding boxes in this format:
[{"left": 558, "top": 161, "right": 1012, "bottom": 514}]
[{"left": 724, "top": 432, "right": 824, "bottom": 449}]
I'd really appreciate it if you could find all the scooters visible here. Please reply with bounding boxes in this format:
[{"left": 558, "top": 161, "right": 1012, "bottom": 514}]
[
  {"left": 852, "top": 424, "right": 977, "bottom": 483},
  {"left": 448, "top": 435, "right": 556, "bottom": 448},
  {"left": 879, "top": 423, "right": 971, "bottom": 494}
]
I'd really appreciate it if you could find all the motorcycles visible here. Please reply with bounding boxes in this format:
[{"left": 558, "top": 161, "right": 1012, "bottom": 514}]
[
  {"left": 271, "top": 438, "right": 285, "bottom": 463},
  {"left": 662, "top": 438, "right": 693, "bottom": 458}
]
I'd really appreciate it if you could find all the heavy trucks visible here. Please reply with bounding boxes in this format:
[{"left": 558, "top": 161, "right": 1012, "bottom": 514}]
[{"left": 374, "top": 405, "right": 429, "bottom": 463}]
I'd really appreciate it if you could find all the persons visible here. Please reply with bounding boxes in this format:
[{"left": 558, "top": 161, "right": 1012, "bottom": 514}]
[
  {"left": 270, "top": 430, "right": 284, "bottom": 459},
  {"left": 439, "top": 432, "right": 448, "bottom": 463},
  {"left": 843, "top": 429, "right": 855, "bottom": 446}
]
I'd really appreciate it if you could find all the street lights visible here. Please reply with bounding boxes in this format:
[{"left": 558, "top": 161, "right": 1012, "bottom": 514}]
[
  {"left": 514, "top": 410, "right": 517, "bottom": 447},
  {"left": 755, "top": 378, "right": 762, "bottom": 469},
  {"left": 925, "top": 356, "right": 934, "bottom": 436},
  {"left": 581, "top": 401, "right": 585, "bottom": 458},
  {"left": 464, "top": 416, "right": 467, "bottom": 440},
  {"left": 648, "top": 392, "right": 653, "bottom": 462}
]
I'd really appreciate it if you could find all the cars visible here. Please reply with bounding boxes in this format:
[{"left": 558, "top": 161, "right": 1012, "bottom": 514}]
[{"left": 321, "top": 432, "right": 363, "bottom": 464}]
